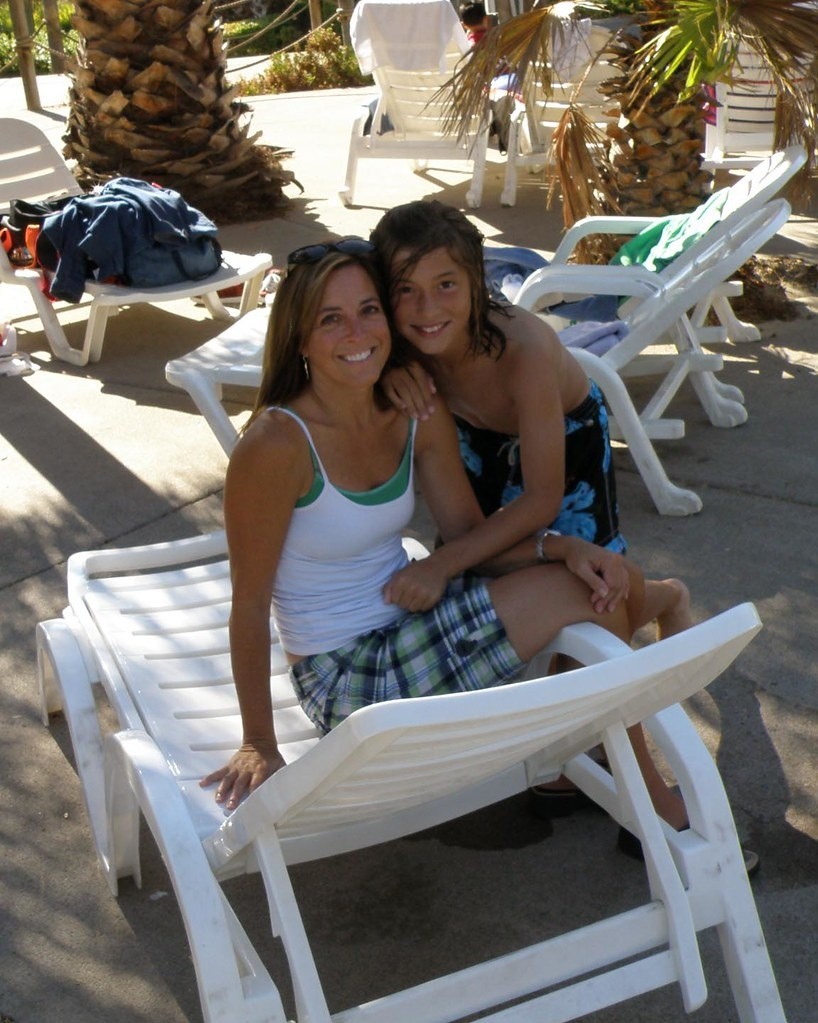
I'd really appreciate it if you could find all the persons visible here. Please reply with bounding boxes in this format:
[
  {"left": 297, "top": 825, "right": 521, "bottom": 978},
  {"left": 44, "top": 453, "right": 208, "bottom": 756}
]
[
  {"left": 199, "top": 237, "right": 760, "bottom": 882},
  {"left": 458, "top": 1, "right": 524, "bottom": 101},
  {"left": 366, "top": 199, "right": 695, "bottom": 645}
]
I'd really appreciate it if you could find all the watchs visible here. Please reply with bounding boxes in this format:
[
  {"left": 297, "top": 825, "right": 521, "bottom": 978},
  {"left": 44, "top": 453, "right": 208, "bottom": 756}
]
[{"left": 535, "top": 530, "right": 561, "bottom": 563}]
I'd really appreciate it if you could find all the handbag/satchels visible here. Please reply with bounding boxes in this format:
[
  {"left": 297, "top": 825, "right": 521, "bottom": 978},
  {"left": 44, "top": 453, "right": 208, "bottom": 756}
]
[
  {"left": 1, "top": 196, "right": 78, "bottom": 268},
  {"left": 111, "top": 227, "right": 223, "bottom": 289}
]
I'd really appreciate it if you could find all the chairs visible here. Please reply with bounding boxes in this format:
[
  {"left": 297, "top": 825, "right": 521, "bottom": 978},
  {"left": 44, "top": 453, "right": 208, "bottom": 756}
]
[
  {"left": 499, "top": 22, "right": 629, "bottom": 206},
  {"left": 506, "top": 145, "right": 809, "bottom": 402},
  {"left": 35, "top": 529, "right": 787, "bottom": 1023},
  {"left": 698, "top": 34, "right": 818, "bottom": 193},
  {"left": 339, "top": 0, "right": 531, "bottom": 207},
  {"left": 162, "top": 199, "right": 793, "bottom": 514},
  {"left": 0, "top": 117, "right": 273, "bottom": 365}
]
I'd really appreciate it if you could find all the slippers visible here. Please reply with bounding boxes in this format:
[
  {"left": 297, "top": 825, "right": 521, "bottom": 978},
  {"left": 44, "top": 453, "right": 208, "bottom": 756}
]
[{"left": 616, "top": 822, "right": 760, "bottom": 873}]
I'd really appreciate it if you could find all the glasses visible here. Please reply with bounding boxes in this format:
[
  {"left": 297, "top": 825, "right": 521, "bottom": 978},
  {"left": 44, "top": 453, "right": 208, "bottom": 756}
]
[{"left": 286, "top": 237, "right": 377, "bottom": 266}]
